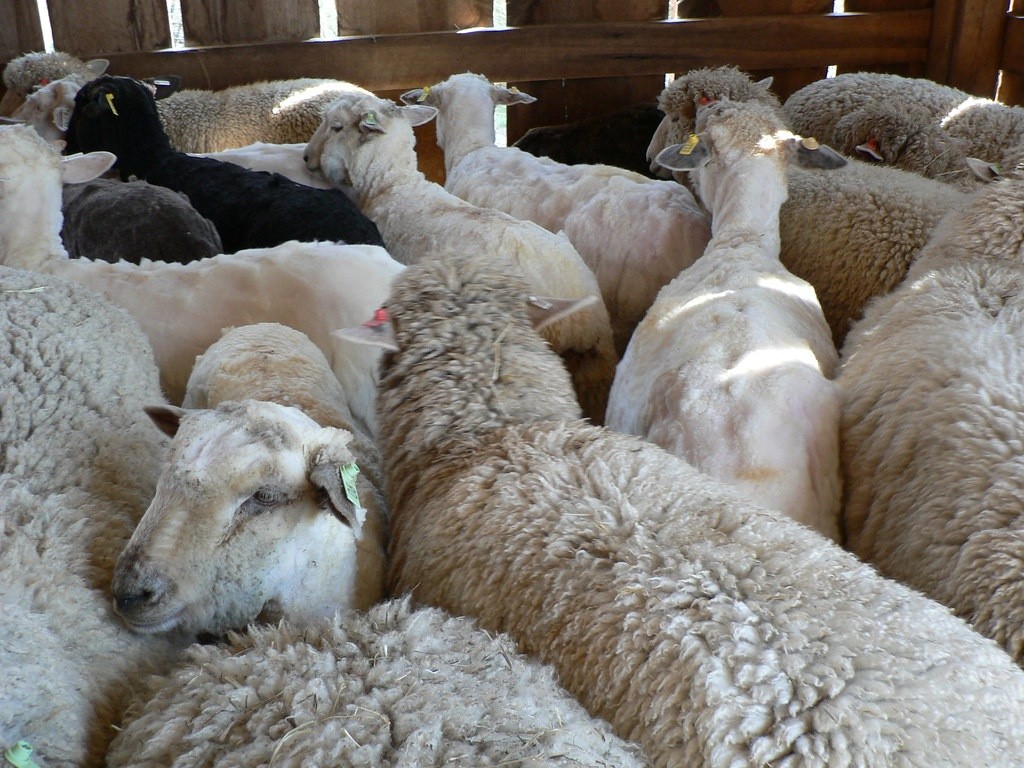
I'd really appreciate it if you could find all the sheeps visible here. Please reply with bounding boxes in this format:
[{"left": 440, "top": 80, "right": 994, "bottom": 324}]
[{"left": 0, "top": 42, "right": 1024, "bottom": 768}]
